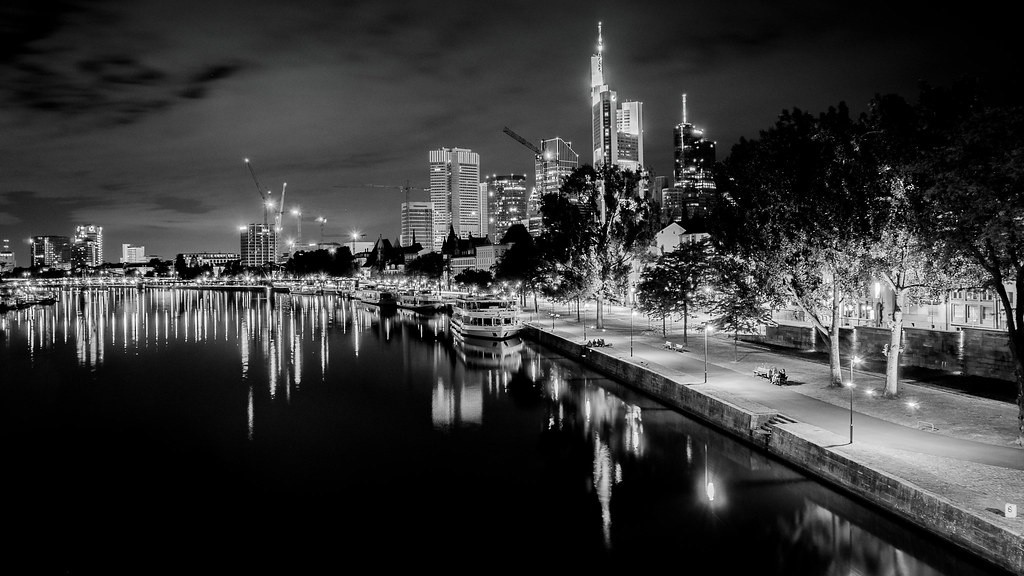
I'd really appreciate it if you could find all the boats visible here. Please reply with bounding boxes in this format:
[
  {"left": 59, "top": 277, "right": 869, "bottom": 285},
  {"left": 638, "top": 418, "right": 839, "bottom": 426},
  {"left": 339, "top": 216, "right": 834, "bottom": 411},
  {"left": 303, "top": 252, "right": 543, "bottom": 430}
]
[
  {"left": 395, "top": 306, "right": 441, "bottom": 321},
  {"left": 451, "top": 332, "right": 527, "bottom": 373},
  {"left": 394, "top": 286, "right": 444, "bottom": 312},
  {"left": 448, "top": 286, "right": 525, "bottom": 339}
]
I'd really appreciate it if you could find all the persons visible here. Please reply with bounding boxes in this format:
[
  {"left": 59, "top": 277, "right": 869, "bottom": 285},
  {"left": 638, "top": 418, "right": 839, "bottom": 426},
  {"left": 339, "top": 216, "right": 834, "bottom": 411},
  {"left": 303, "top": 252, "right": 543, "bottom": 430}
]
[
  {"left": 588, "top": 338, "right": 604, "bottom": 347},
  {"left": 769, "top": 367, "right": 785, "bottom": 385}
]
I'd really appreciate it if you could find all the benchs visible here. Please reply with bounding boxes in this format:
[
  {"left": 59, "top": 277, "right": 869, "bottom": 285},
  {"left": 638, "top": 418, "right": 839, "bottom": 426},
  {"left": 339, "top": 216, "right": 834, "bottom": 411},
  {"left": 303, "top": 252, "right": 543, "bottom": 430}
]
[
  {"left": 754, "top": 367, "right": 771, "bottom": 379},
  {"left": 663, "top": 341, "right": 672, "bottom": 350},
  {"left": 673, "top": 344, "right": 683, "bottom": 353}
]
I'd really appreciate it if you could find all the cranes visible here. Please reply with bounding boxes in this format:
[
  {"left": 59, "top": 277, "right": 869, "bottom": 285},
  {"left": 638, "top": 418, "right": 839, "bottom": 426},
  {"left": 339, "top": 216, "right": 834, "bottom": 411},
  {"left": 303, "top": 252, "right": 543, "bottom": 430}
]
[
  {"left": 322, "top": 229, "right": 367, "bottom": 255},
  {"left": 502, "top": 126, "right": 550, "bottom": 196},
  {"left": 245, "top": 157, "right": 273, "bottom": 223},
  {"left": 330, "top": 176, "right": 433, "bottom": 247}
]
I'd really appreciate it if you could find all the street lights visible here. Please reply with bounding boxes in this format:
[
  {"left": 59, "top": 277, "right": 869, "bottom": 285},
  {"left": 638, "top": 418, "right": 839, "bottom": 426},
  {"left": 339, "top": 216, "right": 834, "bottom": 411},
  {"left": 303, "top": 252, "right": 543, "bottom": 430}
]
[
  {"left": 583, "top": 302, "right": 590, "bottom": 341},
  {"left": 704, "top": 324, "right": 713, "bottom": 383},
  {"left": 848, "top": 353, "right": 861, "bottom": 443},
  {"left": 630, "top": 306, "right": 640, "bottom": 356}
]
